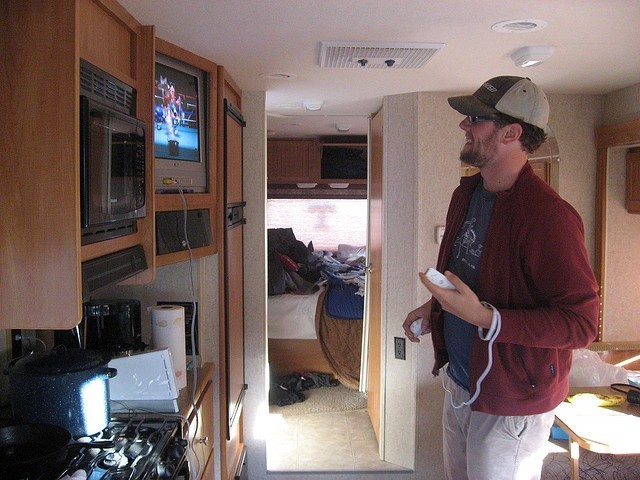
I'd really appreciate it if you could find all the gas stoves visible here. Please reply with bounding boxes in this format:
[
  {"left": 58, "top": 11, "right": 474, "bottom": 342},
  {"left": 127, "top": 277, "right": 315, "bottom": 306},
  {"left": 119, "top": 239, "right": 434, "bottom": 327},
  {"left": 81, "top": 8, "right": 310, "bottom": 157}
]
[{"left": 0, "top": 397, "right": 194, "bottom": 480}]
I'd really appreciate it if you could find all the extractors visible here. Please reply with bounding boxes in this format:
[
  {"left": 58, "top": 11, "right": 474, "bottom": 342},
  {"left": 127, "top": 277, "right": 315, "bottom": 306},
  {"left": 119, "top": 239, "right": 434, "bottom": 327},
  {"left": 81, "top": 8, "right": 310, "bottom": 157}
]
[{"left": 82, "top": 244, "right": 149, "bottom": 298}]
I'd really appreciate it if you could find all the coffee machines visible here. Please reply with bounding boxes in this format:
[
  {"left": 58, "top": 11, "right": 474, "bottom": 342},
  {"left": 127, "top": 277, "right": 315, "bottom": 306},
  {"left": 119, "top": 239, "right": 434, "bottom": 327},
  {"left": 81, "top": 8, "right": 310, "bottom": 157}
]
[{"left": 54, "top": 297, "right": 150, "bottom": 355}]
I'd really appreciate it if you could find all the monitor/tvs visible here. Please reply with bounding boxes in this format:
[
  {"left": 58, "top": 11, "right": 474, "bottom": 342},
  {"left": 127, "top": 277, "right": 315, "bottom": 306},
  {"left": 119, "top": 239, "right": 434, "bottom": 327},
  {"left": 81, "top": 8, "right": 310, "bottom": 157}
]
[{"left": 154, "top": 51, "right": 208, "bottom": 194}]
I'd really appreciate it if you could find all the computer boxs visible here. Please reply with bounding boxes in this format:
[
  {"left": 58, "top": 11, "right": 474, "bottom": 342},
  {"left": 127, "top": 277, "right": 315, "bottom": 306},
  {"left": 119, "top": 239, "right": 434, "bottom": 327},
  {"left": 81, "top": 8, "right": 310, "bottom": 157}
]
[{"left": 155, "top": 209, "right": 213, "bottom": 257}]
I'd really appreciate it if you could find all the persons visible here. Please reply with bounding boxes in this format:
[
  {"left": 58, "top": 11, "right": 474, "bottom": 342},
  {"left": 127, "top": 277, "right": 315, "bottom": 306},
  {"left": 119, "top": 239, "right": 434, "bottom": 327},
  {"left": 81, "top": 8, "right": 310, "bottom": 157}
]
[{"left": 403, "top": 76, "right": 600, "bottom": 480}]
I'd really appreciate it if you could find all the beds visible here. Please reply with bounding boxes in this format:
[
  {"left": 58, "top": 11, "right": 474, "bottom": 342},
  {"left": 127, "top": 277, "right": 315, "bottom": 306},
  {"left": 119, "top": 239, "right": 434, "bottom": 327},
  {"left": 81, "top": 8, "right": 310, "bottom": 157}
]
[{"left": 265, "top": 249, "right": 368, "bottom": 381}]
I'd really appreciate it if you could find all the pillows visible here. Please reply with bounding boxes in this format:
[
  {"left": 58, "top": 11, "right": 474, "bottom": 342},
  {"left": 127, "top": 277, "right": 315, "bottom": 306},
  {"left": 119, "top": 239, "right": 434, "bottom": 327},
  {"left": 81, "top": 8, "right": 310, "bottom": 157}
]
[{"left": 336, "top": 244, "right": 368, "bottom": 263}]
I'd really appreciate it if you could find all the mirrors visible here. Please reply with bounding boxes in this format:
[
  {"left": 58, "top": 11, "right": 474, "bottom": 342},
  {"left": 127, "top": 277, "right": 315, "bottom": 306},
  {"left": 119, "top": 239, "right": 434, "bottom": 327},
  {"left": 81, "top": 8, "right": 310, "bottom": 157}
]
[{"left": 461, "top": 123, "right": 560, "bottom": 197}]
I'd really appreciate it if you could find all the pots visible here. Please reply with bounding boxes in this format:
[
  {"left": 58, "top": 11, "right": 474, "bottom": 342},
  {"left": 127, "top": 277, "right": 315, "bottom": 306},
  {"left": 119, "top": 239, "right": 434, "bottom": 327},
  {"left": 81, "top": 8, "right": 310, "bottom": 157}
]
[
  {"left": 0, "top": 422, "right": 115, "bottom": 480},
  {"left": 0, "top": 344, "right": 116, "bottom": 437}
]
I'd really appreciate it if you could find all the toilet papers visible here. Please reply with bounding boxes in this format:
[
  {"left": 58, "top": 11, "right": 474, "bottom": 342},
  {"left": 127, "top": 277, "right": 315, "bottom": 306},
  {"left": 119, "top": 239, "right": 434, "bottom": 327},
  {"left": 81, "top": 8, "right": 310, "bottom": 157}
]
[{"left": 147, "top": 304, "right": 188, "bottom": 391}]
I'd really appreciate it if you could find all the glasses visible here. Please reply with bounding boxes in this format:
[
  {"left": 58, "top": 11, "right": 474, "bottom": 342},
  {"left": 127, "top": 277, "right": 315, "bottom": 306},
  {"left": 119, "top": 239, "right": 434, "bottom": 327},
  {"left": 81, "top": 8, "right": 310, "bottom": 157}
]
[{"left": 469, "top": 115, "right": 498, "bottom": 124}]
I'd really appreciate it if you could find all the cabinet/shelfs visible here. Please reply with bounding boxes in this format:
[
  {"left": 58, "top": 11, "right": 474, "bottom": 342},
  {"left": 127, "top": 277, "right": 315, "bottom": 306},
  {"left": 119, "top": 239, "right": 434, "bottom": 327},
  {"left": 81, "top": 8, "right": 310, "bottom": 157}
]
[
  {"left": 266, "top": 138, "right": 367, "bottom": 199},
  {"left": 180, "top": 360, "right": 216, "bottom": 480},
  {"left": 217, "top": 64, "right": 246, "bottom": 479},
  {"left": 115, "top": 56, "right": 154, "bottom": 290},
  {"left": 154, "top": 56, "right": 217, "bottom": 266}
]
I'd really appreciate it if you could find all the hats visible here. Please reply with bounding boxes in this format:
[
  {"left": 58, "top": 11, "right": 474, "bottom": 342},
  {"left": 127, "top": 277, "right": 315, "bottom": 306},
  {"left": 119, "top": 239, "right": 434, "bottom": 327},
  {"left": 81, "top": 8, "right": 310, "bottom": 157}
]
[{"left": 448, "top": 75, "right": 550, "bottom": 130}]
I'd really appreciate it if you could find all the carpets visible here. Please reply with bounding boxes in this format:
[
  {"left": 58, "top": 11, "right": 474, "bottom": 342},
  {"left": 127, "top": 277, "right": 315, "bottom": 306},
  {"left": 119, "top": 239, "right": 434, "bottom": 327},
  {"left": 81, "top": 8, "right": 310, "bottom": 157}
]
[{"left": 268, "top": 383, "right": 366, "bottom": 415}]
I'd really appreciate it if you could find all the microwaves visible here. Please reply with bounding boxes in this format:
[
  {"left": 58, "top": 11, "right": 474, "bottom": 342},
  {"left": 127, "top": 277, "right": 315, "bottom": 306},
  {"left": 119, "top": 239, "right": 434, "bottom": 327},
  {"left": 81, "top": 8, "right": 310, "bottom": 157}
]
[{"left": 81, "top": 55, "right": 146, "bottom": 244}]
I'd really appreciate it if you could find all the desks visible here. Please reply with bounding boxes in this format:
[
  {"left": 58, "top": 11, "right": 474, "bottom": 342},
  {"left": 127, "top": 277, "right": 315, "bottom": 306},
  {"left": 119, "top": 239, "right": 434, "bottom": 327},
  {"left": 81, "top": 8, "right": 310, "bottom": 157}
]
[{"left": 554, "top": 385, "right": 640, "bottom": 480}]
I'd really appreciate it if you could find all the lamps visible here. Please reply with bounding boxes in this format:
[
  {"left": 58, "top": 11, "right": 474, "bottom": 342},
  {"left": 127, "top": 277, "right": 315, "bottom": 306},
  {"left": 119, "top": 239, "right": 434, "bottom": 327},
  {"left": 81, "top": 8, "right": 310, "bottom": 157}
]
[
  {"left": 507, "top": 46, "right": 555, "bottom": 68},
  {"left": 304, "top": 101, "right": 322, "bottom": 110},
  {"left": 335, "top": 123, "right": 354, "bottom": 133}
]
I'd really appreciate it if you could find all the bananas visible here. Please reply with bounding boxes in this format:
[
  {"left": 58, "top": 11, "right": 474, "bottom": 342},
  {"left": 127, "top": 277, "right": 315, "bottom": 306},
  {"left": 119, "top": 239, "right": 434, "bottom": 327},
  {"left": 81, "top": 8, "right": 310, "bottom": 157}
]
[{"left": 566, "top": 393, "right": 627, "bottom": 407}]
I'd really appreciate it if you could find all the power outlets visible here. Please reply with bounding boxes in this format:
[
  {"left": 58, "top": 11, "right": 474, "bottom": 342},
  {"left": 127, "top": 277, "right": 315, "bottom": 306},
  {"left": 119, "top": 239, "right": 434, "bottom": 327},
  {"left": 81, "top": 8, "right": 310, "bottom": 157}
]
[{"left": 394, "top": 337, "right": 405, "bottom": 359}]
